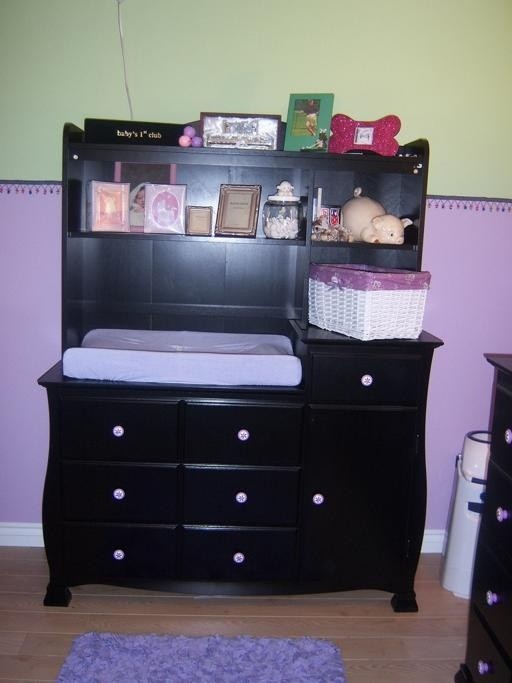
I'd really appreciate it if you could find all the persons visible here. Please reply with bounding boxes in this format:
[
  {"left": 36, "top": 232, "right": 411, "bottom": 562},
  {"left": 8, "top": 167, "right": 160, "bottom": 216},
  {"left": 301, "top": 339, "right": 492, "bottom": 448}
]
[
  {"left": 296, "top": 99, "right": 319, "bottom": 136},
  {"left": 129, "top": 181, "right": 149, "bottom": 227}
]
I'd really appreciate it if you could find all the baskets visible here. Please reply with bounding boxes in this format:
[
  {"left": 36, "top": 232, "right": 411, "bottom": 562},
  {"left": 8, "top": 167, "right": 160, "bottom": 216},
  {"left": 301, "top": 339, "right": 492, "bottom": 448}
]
[{"left": 306, "top": 261, "right": 432, "bottom": 343}]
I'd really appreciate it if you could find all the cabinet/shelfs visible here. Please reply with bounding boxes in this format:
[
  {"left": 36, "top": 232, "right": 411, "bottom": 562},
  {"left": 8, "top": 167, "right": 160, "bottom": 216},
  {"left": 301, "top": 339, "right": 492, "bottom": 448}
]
[
  {"left": 453, "top": 352, "right": 512, "bottom": 683},
  {"left": 36, "top": 120, "right": 444, "bottom": 615}
]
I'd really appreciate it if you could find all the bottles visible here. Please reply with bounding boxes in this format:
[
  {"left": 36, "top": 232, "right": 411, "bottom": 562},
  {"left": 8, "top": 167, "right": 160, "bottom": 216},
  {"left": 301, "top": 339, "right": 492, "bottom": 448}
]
[{"left": 262, "top": 180, "right": 304, "bottom": 240}]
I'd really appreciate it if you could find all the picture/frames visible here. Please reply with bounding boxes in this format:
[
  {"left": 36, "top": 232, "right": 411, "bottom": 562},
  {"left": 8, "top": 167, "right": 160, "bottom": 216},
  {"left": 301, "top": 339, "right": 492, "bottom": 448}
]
[
  {"left": 320, "top": 203, "right": 341, "bottom": 228},
  {"left": 282, "top": 92, "right": 335, "bottom": 155},
  {"left": 212, "top": 184, "right": 263, "bottom": 238},
  {"left": 144, "top": 182, "right": 186, "bottom": 234},
  {"left": 328, "top": 113, "right": 401, "bottom": 157},
  {"left": 88, "top": 180, "right": 131, "bottom": 233},
  {"left": 199, "top": 111, "right": 283, "bottom": 153},
  {"left": 184, "top": 204, "right": 213, "bottom": 236},
  {"left": 114, "top": 160, "right": 178, "bottom": 234}
]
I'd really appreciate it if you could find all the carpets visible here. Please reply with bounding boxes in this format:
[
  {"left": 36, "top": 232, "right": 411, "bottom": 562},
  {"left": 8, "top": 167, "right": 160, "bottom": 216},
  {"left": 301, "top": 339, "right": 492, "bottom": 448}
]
[{"left": 60, "top": 629, "right": 351, "bottom": 683}]
